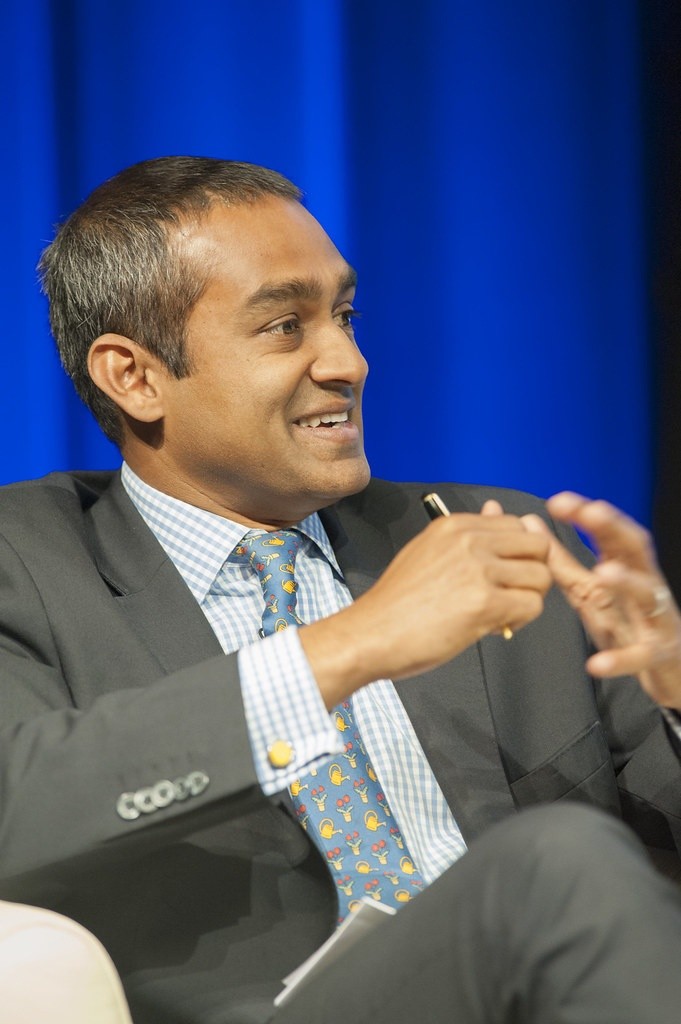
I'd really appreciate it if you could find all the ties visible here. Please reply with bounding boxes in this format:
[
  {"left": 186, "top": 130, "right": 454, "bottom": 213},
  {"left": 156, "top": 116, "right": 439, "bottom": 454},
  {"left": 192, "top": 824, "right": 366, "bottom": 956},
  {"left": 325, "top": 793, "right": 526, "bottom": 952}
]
[{"left": 226, "top": 526, "right": 428, "bottom": 930}]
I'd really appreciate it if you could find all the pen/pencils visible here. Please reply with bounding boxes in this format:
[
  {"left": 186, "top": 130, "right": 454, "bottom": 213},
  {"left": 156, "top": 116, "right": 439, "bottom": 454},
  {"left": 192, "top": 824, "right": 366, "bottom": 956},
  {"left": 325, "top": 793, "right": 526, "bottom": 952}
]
[{"left": 421, "top": 491, "right": 512, "bottom": 640}]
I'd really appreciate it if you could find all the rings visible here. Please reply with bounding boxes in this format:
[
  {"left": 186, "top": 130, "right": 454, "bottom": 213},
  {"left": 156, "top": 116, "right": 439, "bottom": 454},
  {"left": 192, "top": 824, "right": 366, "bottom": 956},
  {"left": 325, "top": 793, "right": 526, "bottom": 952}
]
[{"left": 650, "top": 585, "right": 670, "bottom": 617}]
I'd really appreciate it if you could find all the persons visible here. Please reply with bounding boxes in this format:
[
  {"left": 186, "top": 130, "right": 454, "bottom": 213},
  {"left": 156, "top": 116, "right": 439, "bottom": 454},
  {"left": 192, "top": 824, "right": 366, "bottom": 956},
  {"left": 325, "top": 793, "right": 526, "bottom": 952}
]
[{"left": 0, "top": 154, "right": 681, "bottom": 1024}]
